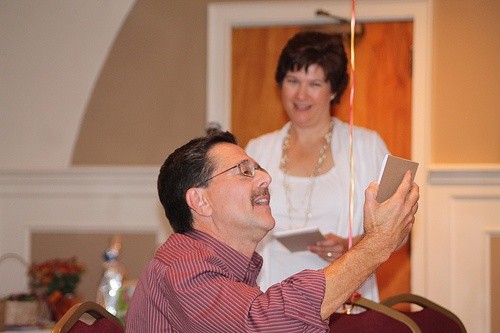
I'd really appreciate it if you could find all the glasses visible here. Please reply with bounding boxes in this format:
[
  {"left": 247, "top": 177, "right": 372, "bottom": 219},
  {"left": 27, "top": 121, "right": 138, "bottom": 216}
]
[{"left": 194, "top": 158, "right": 265, "bottom": 187}]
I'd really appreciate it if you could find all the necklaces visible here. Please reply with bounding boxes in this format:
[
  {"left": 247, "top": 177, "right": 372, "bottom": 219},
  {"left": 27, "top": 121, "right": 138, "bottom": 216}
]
[{"left": 281, "top": 121, "right": 336, "bottom": 228}]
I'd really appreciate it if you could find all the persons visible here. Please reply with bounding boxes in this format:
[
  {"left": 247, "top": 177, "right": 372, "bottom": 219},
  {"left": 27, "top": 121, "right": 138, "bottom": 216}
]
[
  {"left": 245, "top": 31, "right": 392, "bottom": 316},
  {"left": 125, "top": 131, "right": 420, "bottom": 333}
]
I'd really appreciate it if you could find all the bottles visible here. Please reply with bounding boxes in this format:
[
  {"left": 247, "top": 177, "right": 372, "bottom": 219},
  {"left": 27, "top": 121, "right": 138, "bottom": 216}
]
[{"left": 94, "top": 252, "right": 128, "bottom": 315}]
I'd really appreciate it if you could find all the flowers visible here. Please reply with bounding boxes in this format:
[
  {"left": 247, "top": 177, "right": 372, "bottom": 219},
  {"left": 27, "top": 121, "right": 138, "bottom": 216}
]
[{"left": 26, "top": 257, "right": 85, "bottom": 300}]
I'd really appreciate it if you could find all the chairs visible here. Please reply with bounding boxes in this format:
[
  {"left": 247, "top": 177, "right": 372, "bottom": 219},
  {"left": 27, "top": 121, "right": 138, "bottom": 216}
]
[
  {"left": 378, "top": 295, "right": 467, "bottom": 333},
  {"left": 327, "top": 294, "right": 422, "bottom": 333},
  {"left": 51, "top": 301, "right": 125, "bottom": 333}
]
